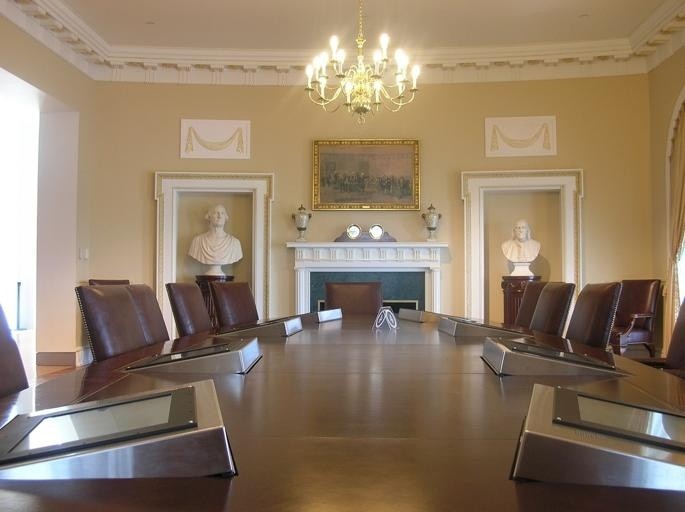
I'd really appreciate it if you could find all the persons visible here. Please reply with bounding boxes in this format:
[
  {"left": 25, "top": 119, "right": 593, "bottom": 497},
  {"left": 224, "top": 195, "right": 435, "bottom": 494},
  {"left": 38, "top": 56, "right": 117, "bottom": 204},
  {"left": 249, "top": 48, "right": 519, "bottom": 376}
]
[
  {"left": 501, "top": 219, "right": 541, "bottom": 262},
  {"left": 321, "top": 157, "right": 411, "bottom": 200},
  {"left": 187, "top": 204, "right": 243, "bottom": 266}
]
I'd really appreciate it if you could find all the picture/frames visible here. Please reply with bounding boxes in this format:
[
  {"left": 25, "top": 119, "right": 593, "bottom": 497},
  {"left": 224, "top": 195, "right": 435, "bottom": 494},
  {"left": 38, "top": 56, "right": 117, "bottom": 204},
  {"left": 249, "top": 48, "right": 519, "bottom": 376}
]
[{"left": 312, "top": 138, "right": 421, "bottom": 212}]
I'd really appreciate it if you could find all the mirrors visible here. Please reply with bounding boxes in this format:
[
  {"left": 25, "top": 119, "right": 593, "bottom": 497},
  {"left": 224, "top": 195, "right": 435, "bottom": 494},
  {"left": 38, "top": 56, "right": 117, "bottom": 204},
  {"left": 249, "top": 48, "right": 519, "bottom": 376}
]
[
  {"left": 369, "top": 223, "right": 384, "bottom": 239},
  {"left": 346, "top": 224, "right": 361, "bottom": 240}
]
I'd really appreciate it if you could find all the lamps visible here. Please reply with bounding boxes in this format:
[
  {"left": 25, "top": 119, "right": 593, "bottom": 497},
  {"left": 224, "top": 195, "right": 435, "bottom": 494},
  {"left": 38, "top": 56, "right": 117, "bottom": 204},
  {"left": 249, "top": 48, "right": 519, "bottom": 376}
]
[
  {"left": 422, "top": 203, "right": 442, "bottom": 241},
  {"left": 291, "top": 204, "right": 312, "bottom": 243},
  {"left": 304, "top": 0, "right": 421, "bottom": 128}
]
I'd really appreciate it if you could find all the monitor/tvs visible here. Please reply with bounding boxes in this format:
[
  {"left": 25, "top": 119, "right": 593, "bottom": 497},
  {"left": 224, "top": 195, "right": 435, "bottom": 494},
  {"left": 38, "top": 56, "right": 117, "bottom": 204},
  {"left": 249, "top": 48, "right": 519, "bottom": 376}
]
[
  {"left": 552, "top": 385, "right": 685, "bottom": 453},
  {"left": 0, "top": 384, "right": 198, "bottom": 467},
  {"left": 126, "top": 344, "right": 230, "bottom": 370},
  {"left": 496, "top": 336, "right": 616, "bottom": 370}
]
